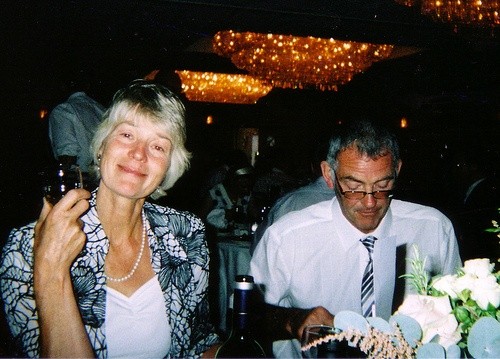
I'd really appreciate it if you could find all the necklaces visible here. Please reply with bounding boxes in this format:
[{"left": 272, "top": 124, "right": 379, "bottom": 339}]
[{"left": 104, "top": 210, "right": 146, "bottom": 281}]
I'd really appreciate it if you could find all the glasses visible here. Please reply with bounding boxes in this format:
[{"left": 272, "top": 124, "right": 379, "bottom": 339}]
[{"left": 333, "top": 170, "right": 397, "bottom": 200}]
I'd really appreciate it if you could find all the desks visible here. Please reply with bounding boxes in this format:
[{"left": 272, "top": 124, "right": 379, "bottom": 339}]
[{"left": 210, "top": 238, "right": 252, "bottom": 329}]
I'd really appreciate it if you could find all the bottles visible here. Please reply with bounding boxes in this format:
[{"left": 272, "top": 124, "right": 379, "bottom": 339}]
[{"left": 213, "top": 275, "right": 269, "bottom": 359}]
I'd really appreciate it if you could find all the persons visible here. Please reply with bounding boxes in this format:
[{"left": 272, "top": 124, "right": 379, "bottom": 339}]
[
  {"left": 0, "top": 73, "right": 222, "bottom": 358},
  {"left": 228, "top": 124, "right": 463, "bottom": 359}
]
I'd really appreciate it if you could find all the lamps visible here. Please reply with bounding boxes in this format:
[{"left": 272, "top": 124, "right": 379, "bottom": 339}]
[
  {"left": 401, "top": 0, "right": 500, "bottom": 32},
  {"left": 146, "top": 70, "right": 273, "bottom": 105},
  {"left": 212, "top": 30, "right": 423, "bottom": 91}
]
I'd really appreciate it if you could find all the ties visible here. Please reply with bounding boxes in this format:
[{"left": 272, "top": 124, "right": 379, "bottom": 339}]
[{"left": 360, "top": 235, "right": 378, "bottom": 317}]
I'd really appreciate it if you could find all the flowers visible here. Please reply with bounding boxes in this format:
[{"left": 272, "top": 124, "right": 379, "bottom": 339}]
[{"left": 301, "top": 209, "right": 500, "bottom": 359}]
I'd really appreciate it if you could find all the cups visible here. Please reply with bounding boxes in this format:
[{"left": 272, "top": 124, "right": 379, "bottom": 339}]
[
  {"left": 301, "top": 324, "right": 347, "bottom": 359},
  {"left": 39, "top": 159, "right": 83, "bottom": 211}
]
